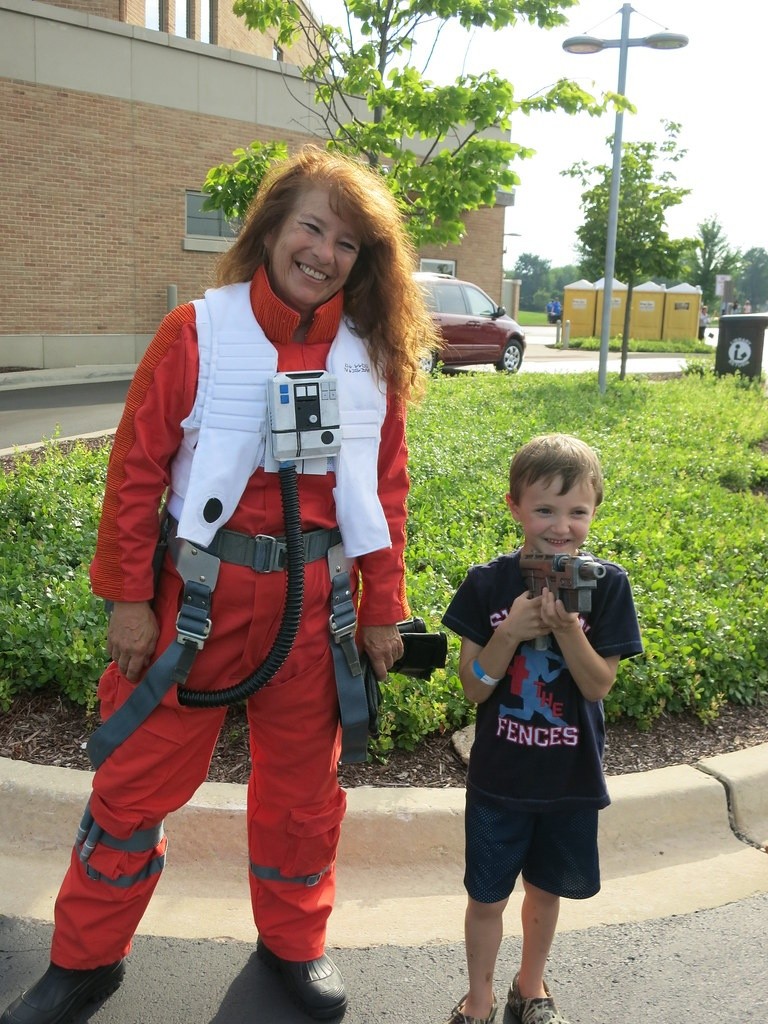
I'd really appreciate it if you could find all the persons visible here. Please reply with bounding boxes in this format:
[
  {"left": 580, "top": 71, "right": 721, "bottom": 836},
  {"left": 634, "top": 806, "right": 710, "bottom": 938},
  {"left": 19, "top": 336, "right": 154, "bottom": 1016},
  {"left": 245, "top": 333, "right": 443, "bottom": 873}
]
[
  {"left": 0, "top": 152, "right": 437, "bottom": 1023},
  {"left": 699, "top": 306, "right": 711, "bottom": 341},
  {"left": 729, "top": 301, "right": 739, "bottom": 314},
  {"left": 546, "top": 298, "right": 562, "bottom": 323},
  {"left": 441, "top": 435, "right": 644, "bottom": 1024},
  {"left": 743, "top": 300, "right": 751, "bottom": 313}
]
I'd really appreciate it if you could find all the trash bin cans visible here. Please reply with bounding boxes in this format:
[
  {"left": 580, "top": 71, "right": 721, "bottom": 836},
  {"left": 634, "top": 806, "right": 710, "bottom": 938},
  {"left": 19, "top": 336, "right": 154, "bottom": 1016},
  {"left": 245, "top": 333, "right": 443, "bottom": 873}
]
[
  {"left": 713, "top": 312, "right": 768, "bottom": 383},
  {"left": 563, "top": 278, "right": 596, "bottom": 338},
  {"left": 664, "top": 283, "right": 703, "bottom": 345},
  {"left": 630, "top": 280, "right": 665, "bottom": 341},
  {"left": 594, "top": 276, "right": 628, "bottom": 338}
]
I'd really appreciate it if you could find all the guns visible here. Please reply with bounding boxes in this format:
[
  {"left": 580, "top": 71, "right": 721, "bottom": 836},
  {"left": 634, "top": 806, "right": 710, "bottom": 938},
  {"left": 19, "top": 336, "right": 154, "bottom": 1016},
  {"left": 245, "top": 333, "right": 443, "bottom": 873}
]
[{"left": 519, "top": 552, "right": 607, "bottom": 653}]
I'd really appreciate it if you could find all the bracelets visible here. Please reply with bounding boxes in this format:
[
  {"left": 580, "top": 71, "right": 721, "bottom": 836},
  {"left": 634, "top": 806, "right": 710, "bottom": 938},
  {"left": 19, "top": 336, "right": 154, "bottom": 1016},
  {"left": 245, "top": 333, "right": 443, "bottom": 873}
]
[{"left": 472, "top": 659, "right": 504, "bottom": 686}]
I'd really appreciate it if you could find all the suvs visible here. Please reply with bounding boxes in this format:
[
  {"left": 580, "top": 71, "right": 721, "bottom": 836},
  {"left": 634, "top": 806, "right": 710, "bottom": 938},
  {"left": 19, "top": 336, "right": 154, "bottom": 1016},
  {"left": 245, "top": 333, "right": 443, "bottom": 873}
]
[{"left": 413, "top": 272, "right": 527, "bottom": 379}]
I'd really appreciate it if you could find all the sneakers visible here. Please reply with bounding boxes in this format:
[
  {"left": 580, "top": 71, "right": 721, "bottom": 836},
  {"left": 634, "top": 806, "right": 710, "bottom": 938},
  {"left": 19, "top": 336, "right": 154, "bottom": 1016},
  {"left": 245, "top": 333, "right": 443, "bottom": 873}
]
[
  {"left": 258, "top": 933, "right": 348, "bottom": 1019},
  {"left": 1, "top": 956, "right": 126, "bottom": 1024}
]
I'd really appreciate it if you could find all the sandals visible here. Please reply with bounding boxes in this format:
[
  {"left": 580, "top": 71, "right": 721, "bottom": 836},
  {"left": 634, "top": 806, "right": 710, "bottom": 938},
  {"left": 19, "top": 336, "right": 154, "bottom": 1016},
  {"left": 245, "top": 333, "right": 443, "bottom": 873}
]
[
  {"left": 508, "top": 970, "right": 569, "bottom": 1024},
  {"left": 446, "top": 990, "right": 499, "bottom": 1024}
]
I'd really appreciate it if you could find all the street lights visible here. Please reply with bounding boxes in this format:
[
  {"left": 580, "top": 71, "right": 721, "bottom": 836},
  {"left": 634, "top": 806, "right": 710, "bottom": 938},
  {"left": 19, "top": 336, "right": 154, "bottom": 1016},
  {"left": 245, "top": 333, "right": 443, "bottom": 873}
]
[{"left": 562, "top": 3, "right": 688, "bottom": 395}]
